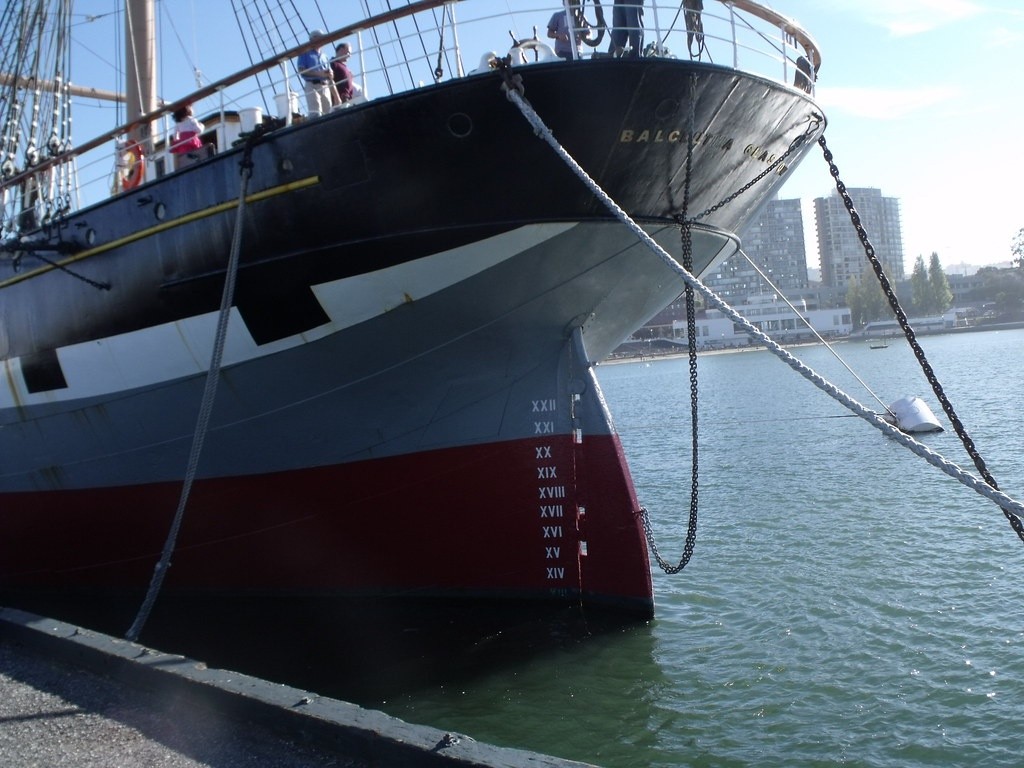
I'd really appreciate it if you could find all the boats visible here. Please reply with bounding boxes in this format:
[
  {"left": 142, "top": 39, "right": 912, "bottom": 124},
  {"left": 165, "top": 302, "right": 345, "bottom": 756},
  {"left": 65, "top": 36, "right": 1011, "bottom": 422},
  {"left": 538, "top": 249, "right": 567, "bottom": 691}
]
[
  {"left": 0, "top": 0, "right": 829, "bottom": 641},
  {"left": 870, "top": 343, "right": 890, "bottom": 349}
]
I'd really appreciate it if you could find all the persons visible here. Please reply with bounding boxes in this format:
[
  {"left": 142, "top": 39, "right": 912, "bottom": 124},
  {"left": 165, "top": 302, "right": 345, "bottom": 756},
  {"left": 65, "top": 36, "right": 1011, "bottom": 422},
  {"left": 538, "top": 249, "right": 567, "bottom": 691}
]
[
  {"left": 170, "top": 106, "right": 204, "bottom": 170},
  {"left": 297, "top": 29, "right": 355, "bottom": 118},
  {"left": 546, "top": 0, "right": 644, "bottom": 62}
]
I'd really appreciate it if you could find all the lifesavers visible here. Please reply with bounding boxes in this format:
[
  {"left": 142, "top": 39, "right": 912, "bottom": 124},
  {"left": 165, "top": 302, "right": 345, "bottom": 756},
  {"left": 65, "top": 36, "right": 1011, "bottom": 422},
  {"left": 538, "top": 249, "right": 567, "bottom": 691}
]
[{"left": 116, "top": 140, "right": 145, "bottom": 191}]
